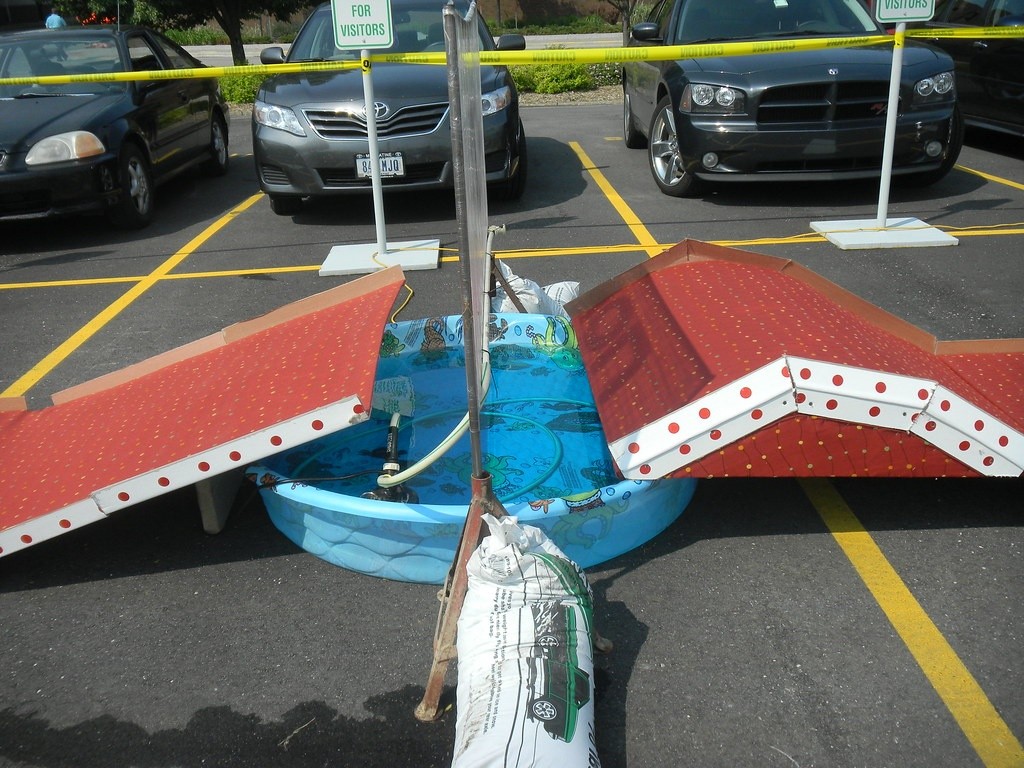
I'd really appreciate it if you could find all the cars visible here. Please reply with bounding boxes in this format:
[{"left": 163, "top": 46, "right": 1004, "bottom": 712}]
[
  {"left": 622, "top": 0, "right": 966, "bottom": 198},
  {"left": 0, "top": 25, "right": 230, "bottom": 231},
  {"left": 252, "top": 0, "right": 526, "bottom": 216}
]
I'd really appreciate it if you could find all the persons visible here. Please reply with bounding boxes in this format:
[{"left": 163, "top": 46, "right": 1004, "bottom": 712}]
[{"left": 46, "top": 8, "right": 68, "bottom": 61}]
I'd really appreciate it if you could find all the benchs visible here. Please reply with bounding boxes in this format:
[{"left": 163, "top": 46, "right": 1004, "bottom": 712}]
[
  {"left": 393, "top": 30, "right": 428, "bottom": 54},
  {"left": 37, "top": 53, "right": 157, "bottom": 87}
]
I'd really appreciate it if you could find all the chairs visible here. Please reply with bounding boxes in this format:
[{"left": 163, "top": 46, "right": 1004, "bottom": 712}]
[{"left": 427, "top": 22, "right": 444, "bottom": 42}]
